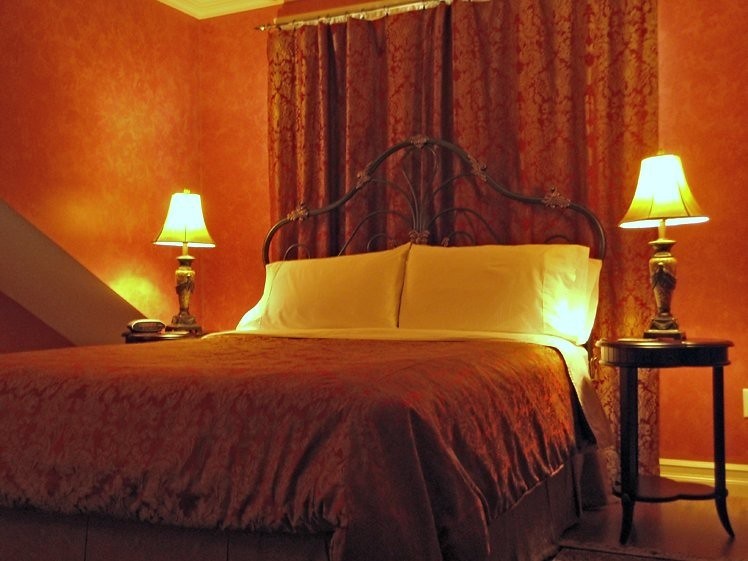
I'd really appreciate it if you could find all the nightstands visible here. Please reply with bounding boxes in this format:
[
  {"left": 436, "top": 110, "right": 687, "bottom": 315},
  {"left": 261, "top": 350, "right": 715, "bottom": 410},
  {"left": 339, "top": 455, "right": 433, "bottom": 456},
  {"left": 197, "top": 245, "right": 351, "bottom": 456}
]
[
  {"left": 594, "top": 339, "right": 735, "bottom": 547},
  {"left": 121, "top": 330, "right": 206, "bottom": 344}
]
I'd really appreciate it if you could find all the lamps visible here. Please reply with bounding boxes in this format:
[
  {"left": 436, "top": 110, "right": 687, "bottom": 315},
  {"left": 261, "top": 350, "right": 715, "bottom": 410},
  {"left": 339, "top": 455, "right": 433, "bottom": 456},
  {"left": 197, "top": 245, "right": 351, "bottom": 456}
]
[
  {"left": 151, "top": 188, "right": 217, "bottom": 334},
  {"left": 617, "top": 149, "right": 710, "bottom": 339}
]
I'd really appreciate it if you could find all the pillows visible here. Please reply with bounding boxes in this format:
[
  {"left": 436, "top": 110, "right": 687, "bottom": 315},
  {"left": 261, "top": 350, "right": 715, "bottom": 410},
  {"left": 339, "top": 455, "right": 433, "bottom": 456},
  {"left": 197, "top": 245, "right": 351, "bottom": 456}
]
[
  {"left": 236, "top": 242, "right": 411, "bottom": 338},
  {"left": 399, "top": 243, "right": 602, "bottom": 346}
]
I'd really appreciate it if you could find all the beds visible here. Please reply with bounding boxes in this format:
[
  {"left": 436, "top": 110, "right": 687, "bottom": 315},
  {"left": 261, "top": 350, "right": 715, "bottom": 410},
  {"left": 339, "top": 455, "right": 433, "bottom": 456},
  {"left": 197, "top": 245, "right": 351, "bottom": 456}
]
[{"left": 0, "top": 133, "right": 618, "bottom": 561}]
